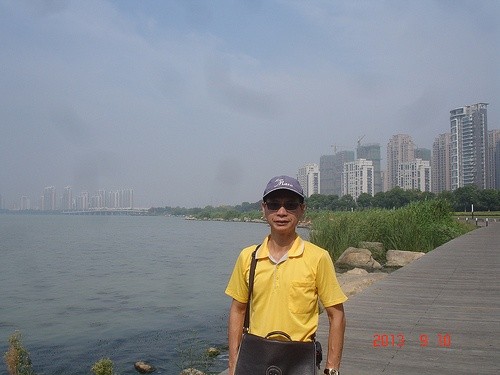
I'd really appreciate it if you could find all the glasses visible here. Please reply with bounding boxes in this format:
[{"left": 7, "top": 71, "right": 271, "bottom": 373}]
[{"left": 263, "top": 201, "right": 304, "bottom": 210}]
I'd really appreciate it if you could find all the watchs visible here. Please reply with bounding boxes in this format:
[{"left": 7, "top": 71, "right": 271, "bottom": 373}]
[{"left": 324, "top": 368, "right": 340, "bottom": 375}]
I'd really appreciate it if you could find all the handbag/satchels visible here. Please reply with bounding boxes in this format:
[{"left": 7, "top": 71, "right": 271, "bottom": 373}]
[{"left": 232, "top": 331, "right": 317, "bottom": 375}]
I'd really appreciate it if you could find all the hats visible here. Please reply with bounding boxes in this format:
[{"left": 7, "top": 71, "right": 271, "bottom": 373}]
[{"left": 262, "top": 175, "right": 305, "bottom": 199}]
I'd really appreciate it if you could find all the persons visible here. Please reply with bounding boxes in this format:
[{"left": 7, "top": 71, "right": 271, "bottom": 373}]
[{"left": 225, "top": 175, "right": 349, "bottom": 375}]
